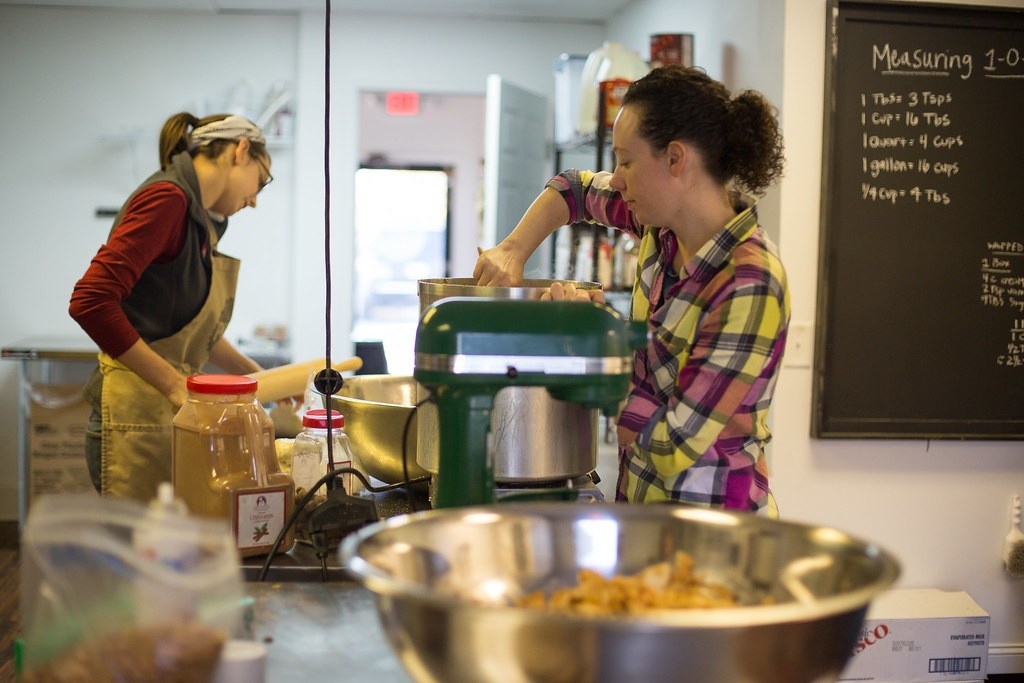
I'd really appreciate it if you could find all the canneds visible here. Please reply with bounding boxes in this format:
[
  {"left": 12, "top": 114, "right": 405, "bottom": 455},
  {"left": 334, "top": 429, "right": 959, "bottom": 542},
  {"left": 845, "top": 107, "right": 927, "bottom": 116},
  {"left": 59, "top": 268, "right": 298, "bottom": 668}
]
[{"left": 649, "top": 33, "right": 694, "bottom": 70}]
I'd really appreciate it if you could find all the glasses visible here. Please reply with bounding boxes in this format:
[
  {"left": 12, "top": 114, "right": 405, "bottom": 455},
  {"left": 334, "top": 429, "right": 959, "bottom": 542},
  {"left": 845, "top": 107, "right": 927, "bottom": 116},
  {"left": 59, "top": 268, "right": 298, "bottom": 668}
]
[{"left": 237, "top": 139, "right": 273, "bottom": 195}]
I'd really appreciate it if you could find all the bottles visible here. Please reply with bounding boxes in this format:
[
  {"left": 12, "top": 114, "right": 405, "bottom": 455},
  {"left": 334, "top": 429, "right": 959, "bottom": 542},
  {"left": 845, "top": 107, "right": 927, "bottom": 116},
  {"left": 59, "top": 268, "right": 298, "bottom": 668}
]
[
  {"left": 169, "top": 373, "right": 296, "bottom": 558},
  {"left": 289, "top": 406, "right": 353, "bottom": 500}
]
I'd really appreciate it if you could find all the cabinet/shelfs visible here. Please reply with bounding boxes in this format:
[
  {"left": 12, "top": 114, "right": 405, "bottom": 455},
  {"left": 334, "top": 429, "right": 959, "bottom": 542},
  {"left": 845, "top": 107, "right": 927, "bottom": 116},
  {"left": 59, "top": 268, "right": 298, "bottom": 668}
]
[{"left": 550, "top": 79, "right": 638, "bottom": 447}]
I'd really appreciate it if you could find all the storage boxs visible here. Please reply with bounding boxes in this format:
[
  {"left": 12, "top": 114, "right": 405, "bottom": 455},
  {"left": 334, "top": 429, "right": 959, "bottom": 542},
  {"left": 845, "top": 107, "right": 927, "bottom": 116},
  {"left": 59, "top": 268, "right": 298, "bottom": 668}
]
[{"left": 837, "top": 588, "right": 992, "bottom": 683}]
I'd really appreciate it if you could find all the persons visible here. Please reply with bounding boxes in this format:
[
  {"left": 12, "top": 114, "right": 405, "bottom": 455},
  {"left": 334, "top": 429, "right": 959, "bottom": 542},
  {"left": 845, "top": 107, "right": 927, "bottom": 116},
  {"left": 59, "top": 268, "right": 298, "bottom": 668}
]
[
  {"left": 68, "top": 112, "right": 272, "bottom": 505},
  {"left": 474, "top": 64, "right": 792, "bottom": 520}
]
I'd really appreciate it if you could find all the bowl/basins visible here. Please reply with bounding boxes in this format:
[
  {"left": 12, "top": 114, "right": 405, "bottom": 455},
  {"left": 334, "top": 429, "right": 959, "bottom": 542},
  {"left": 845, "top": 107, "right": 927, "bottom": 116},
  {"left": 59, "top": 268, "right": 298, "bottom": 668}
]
[{"left": 338, "top": 503, "right": 904, "bottom": 683}]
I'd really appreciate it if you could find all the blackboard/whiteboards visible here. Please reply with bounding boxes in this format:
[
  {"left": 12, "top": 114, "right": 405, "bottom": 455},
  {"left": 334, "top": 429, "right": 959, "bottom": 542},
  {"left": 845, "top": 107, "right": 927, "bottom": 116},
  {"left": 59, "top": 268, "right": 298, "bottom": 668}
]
[{"left": 810, "top": 0, "right": 1024, "bottom": 440}]
